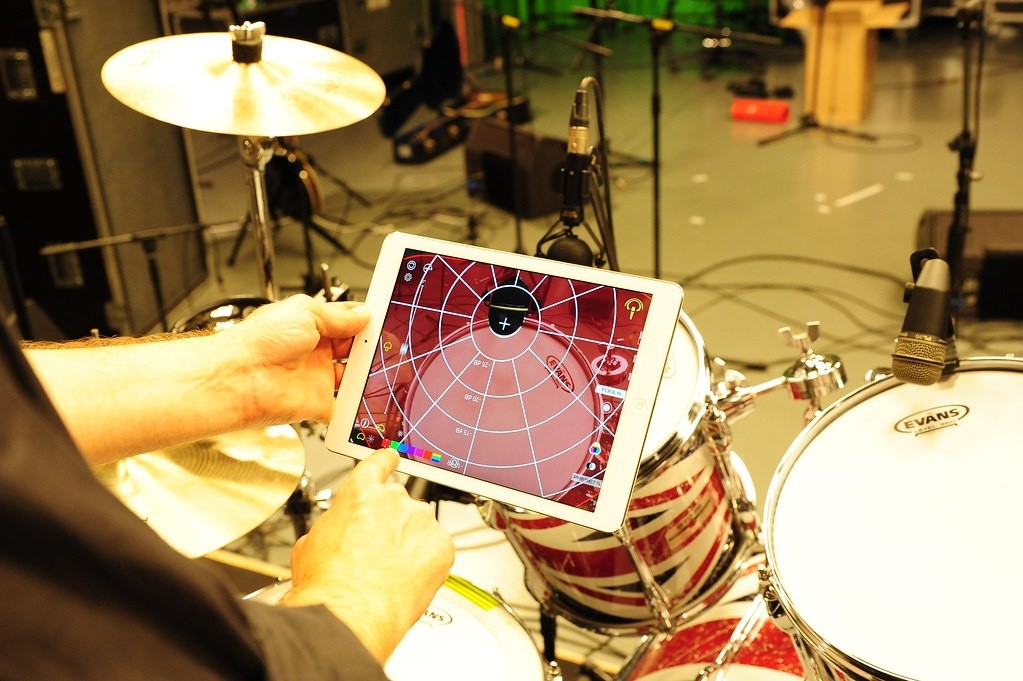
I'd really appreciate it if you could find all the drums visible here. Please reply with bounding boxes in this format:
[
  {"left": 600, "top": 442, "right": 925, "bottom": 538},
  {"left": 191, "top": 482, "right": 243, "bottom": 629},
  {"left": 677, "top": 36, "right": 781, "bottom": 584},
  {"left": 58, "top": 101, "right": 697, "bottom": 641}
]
[
  {"left": 403, "top": 317, "right": 605, "bottom": 503},
  {"left": 493, "top": 303, "right": 745, "bottom": 629},
  {"left": 235, "top": 573, "right": 548, "bottom": 681},
  {"left": 764, "top": 355, "right": 1023, "bottom": 679}
]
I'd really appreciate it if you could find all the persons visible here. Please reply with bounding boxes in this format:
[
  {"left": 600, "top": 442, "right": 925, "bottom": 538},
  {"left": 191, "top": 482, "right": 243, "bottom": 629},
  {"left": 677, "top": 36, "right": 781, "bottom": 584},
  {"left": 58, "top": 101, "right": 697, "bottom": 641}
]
[{"left": 0, "top": 294, "right": 456, "bottom": 681}]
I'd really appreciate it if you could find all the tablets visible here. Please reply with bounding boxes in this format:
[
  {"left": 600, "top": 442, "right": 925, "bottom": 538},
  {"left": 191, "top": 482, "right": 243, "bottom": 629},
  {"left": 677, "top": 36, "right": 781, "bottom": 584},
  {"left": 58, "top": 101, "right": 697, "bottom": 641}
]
[{"left": 323, "top": 232, "right": 684, "bottom": 533}]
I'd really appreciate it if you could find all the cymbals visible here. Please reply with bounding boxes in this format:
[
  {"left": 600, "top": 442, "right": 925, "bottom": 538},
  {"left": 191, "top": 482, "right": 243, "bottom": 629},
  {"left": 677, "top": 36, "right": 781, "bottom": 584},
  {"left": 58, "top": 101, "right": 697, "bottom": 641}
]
[
  {"left": 100, "top": 31, "right": 387, "bottom": 137},
  {"left": 117, "top": 423, "right": 310, "bottom": 559}
]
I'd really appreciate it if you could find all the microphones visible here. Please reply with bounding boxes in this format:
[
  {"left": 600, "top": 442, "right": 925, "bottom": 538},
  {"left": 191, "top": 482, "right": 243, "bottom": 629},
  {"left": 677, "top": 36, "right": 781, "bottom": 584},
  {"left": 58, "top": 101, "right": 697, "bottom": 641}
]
[
  {"left": 890, "top": 247, "right": 960, "bottom": 386},
  {"left": 544, "top": 88, "right": 594, "bottom": 268}
]
